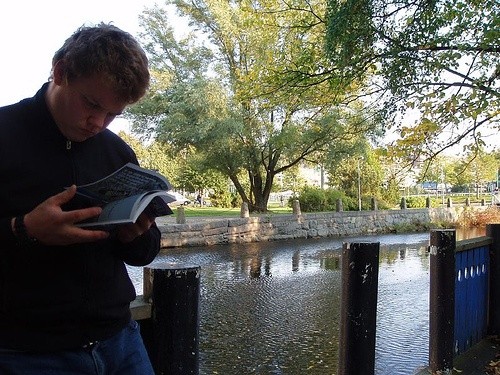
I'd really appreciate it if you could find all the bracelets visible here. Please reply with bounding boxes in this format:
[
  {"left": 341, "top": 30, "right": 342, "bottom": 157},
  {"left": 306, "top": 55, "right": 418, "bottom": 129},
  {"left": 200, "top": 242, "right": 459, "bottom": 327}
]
[{"left": 13, "top": 212, "right": 33, "bottom": 242}]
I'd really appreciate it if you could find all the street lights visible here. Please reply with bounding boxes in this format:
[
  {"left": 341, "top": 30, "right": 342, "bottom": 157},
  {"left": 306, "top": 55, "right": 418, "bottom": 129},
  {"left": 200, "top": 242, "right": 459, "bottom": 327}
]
[{"left": 358, "top": 156, "right": 364, "bottom": 211}]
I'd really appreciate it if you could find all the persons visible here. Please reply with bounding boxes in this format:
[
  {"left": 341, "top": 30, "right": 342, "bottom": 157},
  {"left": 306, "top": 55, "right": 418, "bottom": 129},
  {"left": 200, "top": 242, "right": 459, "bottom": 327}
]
[
  {"left": 0, "top": 23, "right": 162, "bottom": 375},
  {"left": 193, "top": 194, "right": 201, "bottom": 207}
]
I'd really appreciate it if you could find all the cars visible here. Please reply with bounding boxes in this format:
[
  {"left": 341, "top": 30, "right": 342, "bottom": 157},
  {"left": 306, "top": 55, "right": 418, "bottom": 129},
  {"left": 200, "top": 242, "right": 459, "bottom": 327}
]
[{"left": 168, "top": 192, "right": 191, "bottom": 206}]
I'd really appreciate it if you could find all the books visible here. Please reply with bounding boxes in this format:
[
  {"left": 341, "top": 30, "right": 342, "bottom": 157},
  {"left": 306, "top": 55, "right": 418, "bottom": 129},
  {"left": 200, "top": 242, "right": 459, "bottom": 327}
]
[{"left": 62, "top": 161, "right": 177, "bottom": 230}]
